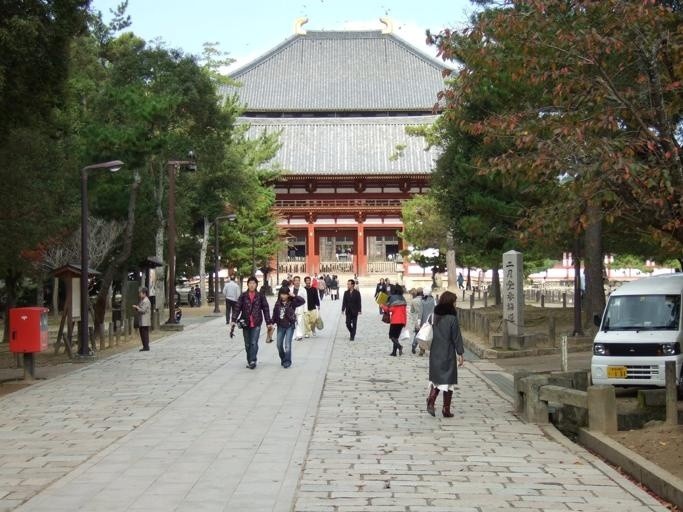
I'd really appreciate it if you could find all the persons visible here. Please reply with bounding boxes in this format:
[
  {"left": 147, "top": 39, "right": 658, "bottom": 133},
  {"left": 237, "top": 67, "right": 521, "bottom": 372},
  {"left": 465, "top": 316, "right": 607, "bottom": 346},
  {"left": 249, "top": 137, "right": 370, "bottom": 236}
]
[
  {"left": 194, "top": 284, "right": 200, "bottom": 307},
  {"left": 131, "top": 286, "right": 150, "bottom": 352},
  {"left": 421, "top": 290, "right": 464, "bottom": 418},
  {"left": 214, "top": 270, "right": 436, "bottom": 369},
  {"left": 457, "top": 272, "right": 463, "bottom": 289},
  {"left": 187, "top": 286, "right": 195, "bottom": 307}
]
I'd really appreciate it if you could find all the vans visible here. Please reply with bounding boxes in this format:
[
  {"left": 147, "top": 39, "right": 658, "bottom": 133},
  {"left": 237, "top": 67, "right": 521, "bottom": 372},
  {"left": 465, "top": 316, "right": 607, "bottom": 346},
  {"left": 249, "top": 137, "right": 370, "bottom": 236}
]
[{"left": 591, "top": 272, "right": 683, "bottom": 393}]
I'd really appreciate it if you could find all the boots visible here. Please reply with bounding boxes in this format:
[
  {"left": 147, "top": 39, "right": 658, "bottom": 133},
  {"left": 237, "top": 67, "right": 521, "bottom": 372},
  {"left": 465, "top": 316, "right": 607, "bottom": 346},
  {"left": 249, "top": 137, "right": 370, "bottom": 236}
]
[
  {"left": 426, "top": 384, "right": 440, "bottom": 416},
  {"left": 390, "top": 344, "right": 397, "bottom": 356},
  {"left": 442, "top": 390, "right": 454, "bottom": 417},
  {"left": 392, "top": 336, "right": 402, "bottom": 355}
]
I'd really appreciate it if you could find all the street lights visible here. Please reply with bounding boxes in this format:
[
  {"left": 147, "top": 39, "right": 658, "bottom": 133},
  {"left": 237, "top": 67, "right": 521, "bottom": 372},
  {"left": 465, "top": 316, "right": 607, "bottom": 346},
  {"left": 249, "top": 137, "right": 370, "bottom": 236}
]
[
  {"left": 276, "top": 240, "right": 290, "bottom": 287},
  {"left": 251, "top": 230, "right": 267, "bottom": 279},
  {"left": 79, "top": 158, "right": 127, "bottom": 358},
  {"left": 558, "top": 172, "right": 584, "bottom": 336},
  {"left": 212, "top": 214, "right": 238, "bottom": 313},
  {"left": 166, "top": 154, "right": 198, "bottom": 324}
]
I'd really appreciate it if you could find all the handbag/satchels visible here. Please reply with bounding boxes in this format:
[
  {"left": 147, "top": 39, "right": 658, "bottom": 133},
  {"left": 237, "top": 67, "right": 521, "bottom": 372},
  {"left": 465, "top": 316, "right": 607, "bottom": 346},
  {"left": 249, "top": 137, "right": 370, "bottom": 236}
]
[
  {"left": 415, "top": 312, "right": 434, "bottom": 351},
  {"left": 279, "top": 319, "right": 290, "bottom": 328},
  {"left": 316, "top": 318, "right": 324, "bottom": 329},
  {"left": 236, "top": 317, "right": 249, "bottom": 328},
  {"left": 134, "top": 313, "right": 142, "bottom": 328},
  {"left": 382, "top": 312, "right": 390, "bottom": 323}
]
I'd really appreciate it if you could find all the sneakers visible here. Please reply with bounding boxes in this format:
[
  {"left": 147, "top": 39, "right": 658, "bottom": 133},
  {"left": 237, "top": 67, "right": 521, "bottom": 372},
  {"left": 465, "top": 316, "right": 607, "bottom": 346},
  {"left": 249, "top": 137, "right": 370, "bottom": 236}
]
[
  {"left": 246, "top": 362, "right": 256, "bottom": 369},
  {"left": 350, "top": 330, "right": 354, "bottom": 340},
  {"left": 313, "top": 332, "right": 316, "bottom": 336},
  {"left": 140, "top": 348, "right": 149, "bottom": 351},
  {"left": 281, "top": 358, "right": 291, "bottom": 368},
  {"left": 305, "top": 335, "right": 310, "bottom": 338},
  {"left": 294, "top": 337, "right": 302, "bottom": 341},
  {"left": 412, "top": 343, "right": 417, "bottom": 354}
]
